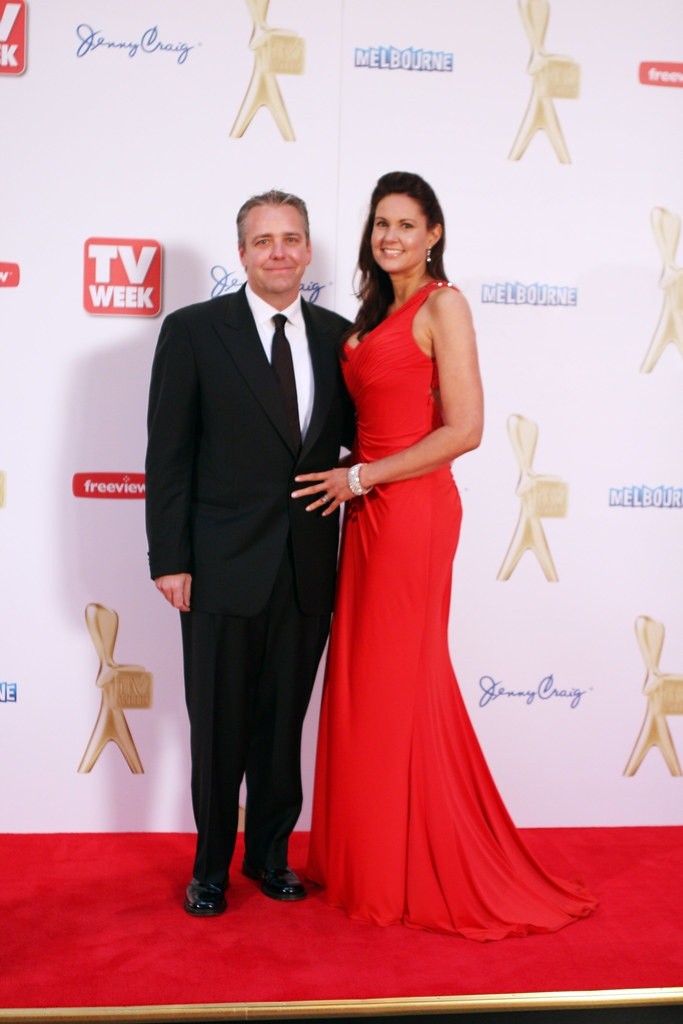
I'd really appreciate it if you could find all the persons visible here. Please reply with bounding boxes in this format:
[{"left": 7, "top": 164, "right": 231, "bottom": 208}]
[
  {"left": 290, "top": 171, "right": 597, "bottom": 942},
  {"left": 145, "top": 190, "right": 357, "bottom": 918}
]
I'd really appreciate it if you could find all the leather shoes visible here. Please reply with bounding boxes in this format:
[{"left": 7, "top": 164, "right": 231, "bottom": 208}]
[
  {"left": 183, "top": 871, "right": 230, "bottom": 916},
  {"left": 242, "top": 859, "right": 307, "bottom": 900}
]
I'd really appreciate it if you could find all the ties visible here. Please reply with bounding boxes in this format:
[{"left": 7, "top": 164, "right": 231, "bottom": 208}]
[{"left": 270, "top": 313, "right": 302, "bottom": 448}]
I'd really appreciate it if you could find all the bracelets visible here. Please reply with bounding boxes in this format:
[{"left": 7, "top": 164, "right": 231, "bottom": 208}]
[{"left": 348, "top": 463, "right": 372, "bottom": 495}]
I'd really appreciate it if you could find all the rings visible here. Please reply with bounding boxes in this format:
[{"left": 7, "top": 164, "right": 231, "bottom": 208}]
[{"left": 322, "top": 495, "right": 328, "bottom": 502}]
